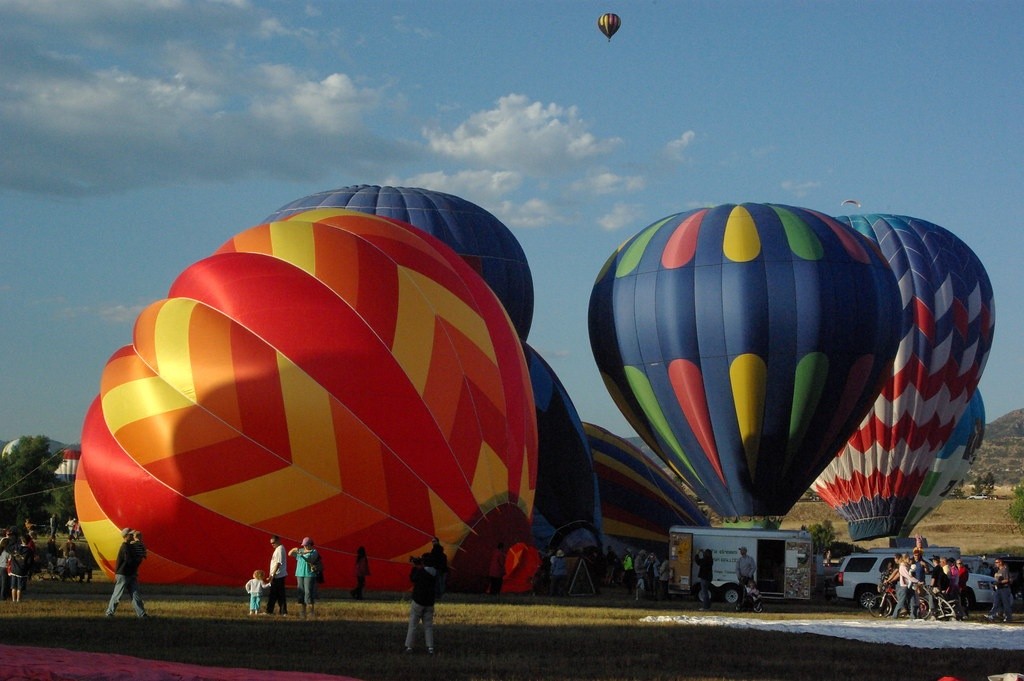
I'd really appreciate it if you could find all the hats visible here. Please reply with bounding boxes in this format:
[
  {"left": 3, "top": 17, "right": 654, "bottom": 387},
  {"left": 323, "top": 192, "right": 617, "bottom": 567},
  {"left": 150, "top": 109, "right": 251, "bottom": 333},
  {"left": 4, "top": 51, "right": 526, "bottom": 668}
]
[
  {"left": 638, "top": 549, "right": 648, "bottom": 557},
  {"left": 301, "top": 538, "right": 312, "bottom": 545},
  {"left": 122, "top": 528, "right": 135, "bottom": 537},
  {"left": 557, "top": 550, "right": 564, "bottom": 557},
  {"left": 739, "top": 547, "right": 747, "bottom": 552}
]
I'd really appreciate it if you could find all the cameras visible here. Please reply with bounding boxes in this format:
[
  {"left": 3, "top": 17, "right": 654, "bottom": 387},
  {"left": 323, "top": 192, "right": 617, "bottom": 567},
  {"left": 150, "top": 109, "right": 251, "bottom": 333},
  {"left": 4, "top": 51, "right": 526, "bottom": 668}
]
[
  {"left": 409, "top": 555, "right": 422, "bottom": 566},
  {"left": 700, "top": 549, "right": 703, "bottom": 551}
]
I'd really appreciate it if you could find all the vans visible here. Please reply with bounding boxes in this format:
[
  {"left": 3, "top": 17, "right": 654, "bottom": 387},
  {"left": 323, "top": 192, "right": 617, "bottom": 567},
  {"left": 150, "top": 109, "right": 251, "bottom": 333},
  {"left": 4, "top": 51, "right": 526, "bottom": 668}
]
[{"left": 834, "top": 552, "right": 996, "bottom": 610}]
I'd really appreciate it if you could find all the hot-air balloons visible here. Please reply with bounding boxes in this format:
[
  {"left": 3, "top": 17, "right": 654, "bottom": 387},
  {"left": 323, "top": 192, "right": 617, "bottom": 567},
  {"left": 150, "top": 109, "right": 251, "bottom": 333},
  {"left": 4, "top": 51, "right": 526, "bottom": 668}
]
[
  {"left": 578, "top": 421, "right": 712, "bottom": 558},
  {"left": 586, "top": 201, "right": 902, "bottom": 530},
  {"left": 887, "top": 386, "right": 986, "bottom": 547},
  {"left": 519, "top": 339, "right": 602, "bottom": 586},
  {"left": 72, "top": 209, "right": 598, "bottom": 598},
  {"left": 597, "top": 12, "right": 622, "bottom": 42},
  {"left": 253, "top": 184, "right": 536, "bottom": 350},
  {"left": 808, "top": 212, "right": 996, "bottom": 542}
]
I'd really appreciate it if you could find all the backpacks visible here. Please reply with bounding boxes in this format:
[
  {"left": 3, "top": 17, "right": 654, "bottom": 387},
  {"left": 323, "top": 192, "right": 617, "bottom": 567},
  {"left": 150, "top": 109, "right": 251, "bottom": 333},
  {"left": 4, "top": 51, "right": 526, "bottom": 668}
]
[
  {"left": 980, "top": 564, "right": 992, "bottom": 576},
  {"left": 938, "top": 572, "right": 950, "bottom": 590}
]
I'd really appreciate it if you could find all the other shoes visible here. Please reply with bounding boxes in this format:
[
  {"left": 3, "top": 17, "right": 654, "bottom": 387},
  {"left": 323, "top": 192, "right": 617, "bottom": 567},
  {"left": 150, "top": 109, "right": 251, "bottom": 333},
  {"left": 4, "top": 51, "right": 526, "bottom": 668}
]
[{"left": 984, "top": 614, "right": 993, "bottom": 619}]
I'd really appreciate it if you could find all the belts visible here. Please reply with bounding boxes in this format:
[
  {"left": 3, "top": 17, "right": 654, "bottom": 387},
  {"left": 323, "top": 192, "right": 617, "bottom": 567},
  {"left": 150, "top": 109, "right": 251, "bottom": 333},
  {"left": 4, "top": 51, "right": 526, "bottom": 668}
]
[{"left": 998, "top": 586, "right": 1008, "bottom": 588}]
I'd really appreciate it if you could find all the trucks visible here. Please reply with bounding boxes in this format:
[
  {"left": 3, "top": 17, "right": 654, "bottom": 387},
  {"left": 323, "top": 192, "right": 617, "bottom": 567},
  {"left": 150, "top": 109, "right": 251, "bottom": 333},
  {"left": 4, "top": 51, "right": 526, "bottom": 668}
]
[
  {"left": 869, "top": 546, "right": 992, "bottom": 576},
  {"left": 668, "top": 525, "right": 841, "bottom": 605}
]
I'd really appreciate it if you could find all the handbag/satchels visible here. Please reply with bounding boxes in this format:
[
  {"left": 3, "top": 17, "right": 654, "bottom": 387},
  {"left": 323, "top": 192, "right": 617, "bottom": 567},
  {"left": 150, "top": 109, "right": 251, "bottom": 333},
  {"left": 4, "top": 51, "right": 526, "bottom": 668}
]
[
  {"left": 309, "top": 571, "right": 324, "bottom": 583},
  {"left": 309, "top": 551, "right": 322, "bottom": 572}
]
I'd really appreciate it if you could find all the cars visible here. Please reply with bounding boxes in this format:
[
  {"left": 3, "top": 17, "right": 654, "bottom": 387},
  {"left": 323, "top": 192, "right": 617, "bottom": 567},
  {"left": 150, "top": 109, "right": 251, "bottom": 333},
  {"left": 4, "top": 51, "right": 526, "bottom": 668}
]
[
  {"left": 968, "top": 493, "right": 991, "bottom": 499},
  {"left": 992, "top": 558, "right": 1024, "bottom": 609}
]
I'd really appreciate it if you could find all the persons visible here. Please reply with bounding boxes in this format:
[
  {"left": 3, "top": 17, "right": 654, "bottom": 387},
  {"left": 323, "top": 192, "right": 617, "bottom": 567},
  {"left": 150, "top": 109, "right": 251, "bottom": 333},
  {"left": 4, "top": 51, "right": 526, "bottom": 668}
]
[
  {"left": 736, "top": 546, "right": 756, "bottom": 612},
  {"left": 105, "top": 527, "right": 150, "bottom": 619},
  {"left": 883, "top": 539, "right": 970, "bottom": 620},
  {"left": 287, "top": 537, "right": 320, "bottom": 618},
  {"left": 582, "top": 545, "right": 620, "bottom": 594},
  {"left": 743, "top": 580, "right": 763, "bottom": 612},
  {"left": 547, "top": 549, "right": 570, "bottom": 598},
  {"left": 621, "top": 546, "right": 669, "bottom": 603},
  {"left": 695, "top": 548, "right": 713, "bottom": 611},
  {"left": 431, "top": 538, "right": 449, "bottom": 601},
  {"left": 489, "top": 542, "right": 506, "bottom": 604},
  {"left": 405, "top": 552, "right": 437, "bottom": 654},
  {"left": 245, "top": 570, "right": 272, "bottom": 616},
  {"left": 258, "top": 535, "right": 288, "bottom": 616},
  {"left": 349, "top": 547, "right": 370, "bottom": 600},
  {"left": 0, "top": 513, "right": 94, "bottom": 602},
  {"left": 984, "top": 558, "right": 1014, "bottom": 623}
]
